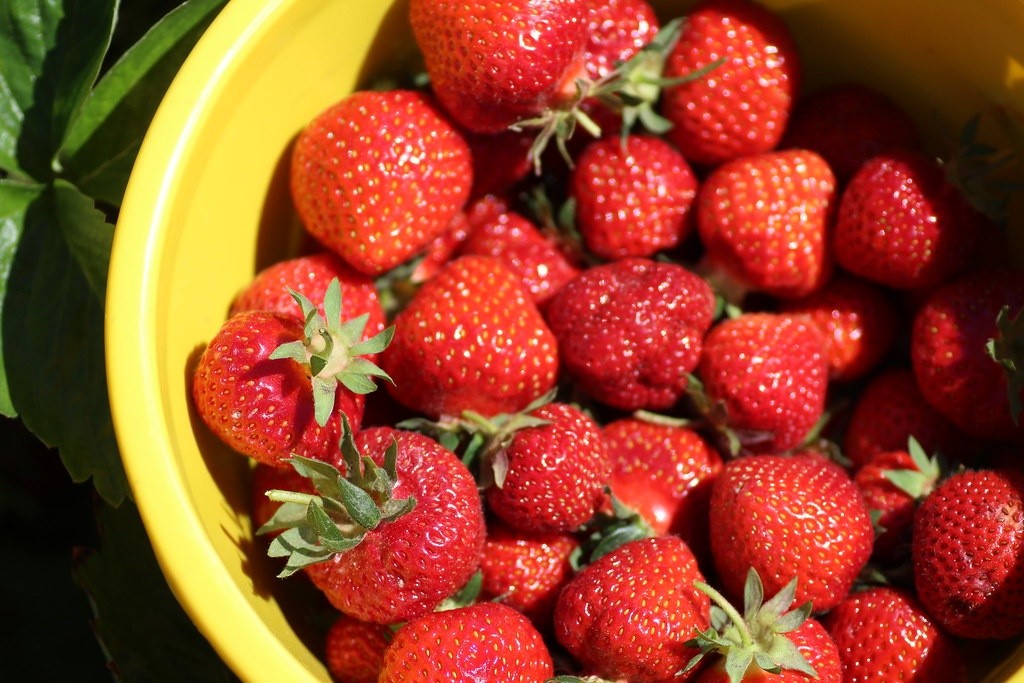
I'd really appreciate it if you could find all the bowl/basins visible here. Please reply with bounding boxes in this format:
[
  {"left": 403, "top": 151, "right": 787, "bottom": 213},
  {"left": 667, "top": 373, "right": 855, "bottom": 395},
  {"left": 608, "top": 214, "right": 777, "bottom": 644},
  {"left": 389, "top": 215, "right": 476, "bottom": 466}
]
[{"left": 98, "top": 1, "right": 1024, "bottom": 683}]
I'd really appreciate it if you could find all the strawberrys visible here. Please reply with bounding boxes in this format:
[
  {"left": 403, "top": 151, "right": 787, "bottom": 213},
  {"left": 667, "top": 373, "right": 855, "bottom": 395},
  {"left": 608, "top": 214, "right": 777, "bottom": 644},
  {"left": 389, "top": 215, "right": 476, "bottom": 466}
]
[{"left": 193, "top": 0, "right": 1024, "bottom": 683}]
